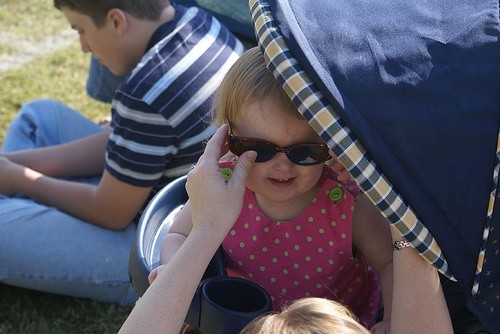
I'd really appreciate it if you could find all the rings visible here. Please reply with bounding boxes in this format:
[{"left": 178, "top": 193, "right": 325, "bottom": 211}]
[{"left": 189, "top": 164, "right": 195, "bottom": 171}]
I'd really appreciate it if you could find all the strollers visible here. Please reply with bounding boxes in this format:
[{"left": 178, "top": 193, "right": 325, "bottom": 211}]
[{"left": 126, "top": 0, "right": 500, "bottom": 334}]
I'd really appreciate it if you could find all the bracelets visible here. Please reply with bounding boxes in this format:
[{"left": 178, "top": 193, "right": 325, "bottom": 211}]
[{"left": 392, "top": 240, "right": 411, "bottom": 250}]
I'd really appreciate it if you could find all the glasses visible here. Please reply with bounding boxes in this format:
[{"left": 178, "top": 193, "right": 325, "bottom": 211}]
[{"left": 226, "top": 117, "right": 333, "bottom": 166}]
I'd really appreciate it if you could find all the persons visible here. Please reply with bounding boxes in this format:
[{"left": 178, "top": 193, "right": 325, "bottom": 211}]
[
  {"left": 117, "top": 124, "right": 455, "bottom": 334},
  {"left": 0, "top": 0, "right": 250, "bottom": 305},
  {"left": 148, "top": 47, "right": 393, "bottom": 334}
]
